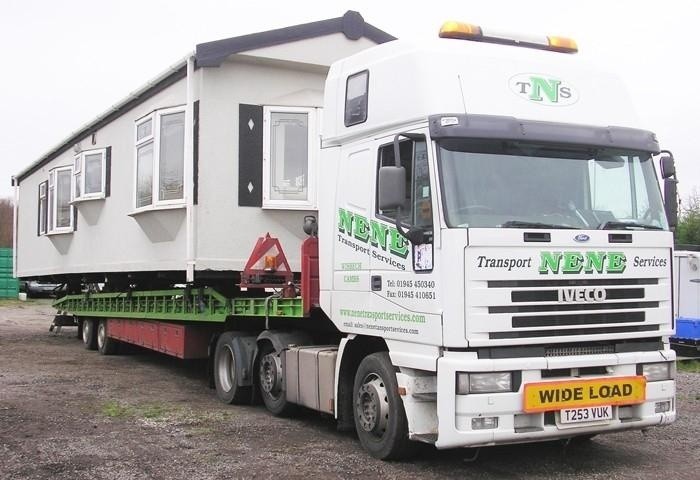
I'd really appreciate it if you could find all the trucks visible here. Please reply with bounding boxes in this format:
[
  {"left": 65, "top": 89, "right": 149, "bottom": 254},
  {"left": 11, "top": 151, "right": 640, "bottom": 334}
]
[
  {"left": 673, "top": 246, "right": 700, "bottom": 354},
  {"left": 9, "top": 10, "right": 678, "bottom": 466}
]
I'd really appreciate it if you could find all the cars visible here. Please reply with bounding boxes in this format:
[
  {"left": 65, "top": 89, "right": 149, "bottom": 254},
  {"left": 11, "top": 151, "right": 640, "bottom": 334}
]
[{"left": 22, "top": 275, "right": 91, "bottom": 295}]
